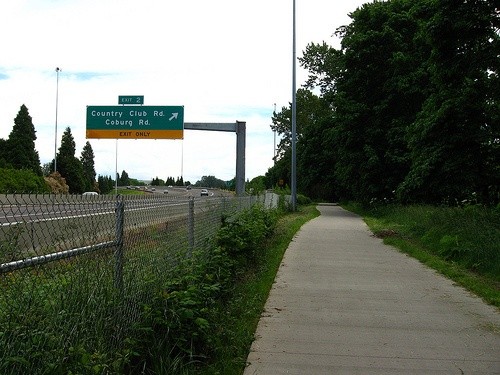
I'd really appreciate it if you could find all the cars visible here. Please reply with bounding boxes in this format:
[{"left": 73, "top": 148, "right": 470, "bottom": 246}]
[
  {"left": 82, "top": 191, "right": 98, "bottom": 196},
  {"left": 125, "top": 184, "right": 192, "bottom": 194}
]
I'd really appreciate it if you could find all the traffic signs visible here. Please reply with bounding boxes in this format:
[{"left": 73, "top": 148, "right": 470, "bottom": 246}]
[{"left": 85, "top": 105, "right": 184, "bottom": 139}]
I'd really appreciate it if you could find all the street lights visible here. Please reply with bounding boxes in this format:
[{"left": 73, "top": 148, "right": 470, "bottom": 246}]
[
  {"left": 274, "top": 102, "right": 277, "bottom": 158},
  {"left": 55, "top": 67, "right": 61, "bottom": 174}
]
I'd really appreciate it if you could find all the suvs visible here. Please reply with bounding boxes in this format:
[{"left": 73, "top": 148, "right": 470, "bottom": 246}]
[{"left": 200, "top": 188, "right": 208, "bottom": 196}]
